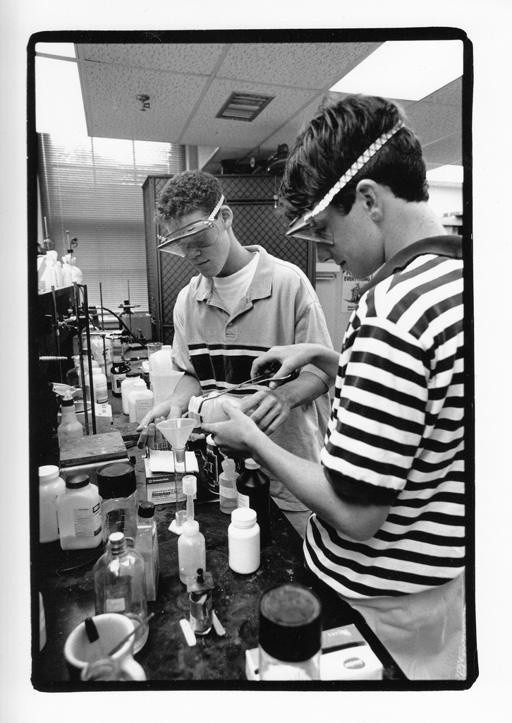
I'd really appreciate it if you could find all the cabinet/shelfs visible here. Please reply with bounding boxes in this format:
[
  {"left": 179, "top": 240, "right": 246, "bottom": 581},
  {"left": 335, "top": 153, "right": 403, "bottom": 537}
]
[{"left": 142, "top": 173, "right": 316, "bottom": 346}]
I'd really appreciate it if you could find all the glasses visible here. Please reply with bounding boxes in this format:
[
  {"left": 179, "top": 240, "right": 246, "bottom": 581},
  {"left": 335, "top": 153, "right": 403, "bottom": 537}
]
[
  {"left": 154, "top": 193, "right": 228, "bottom": 260},
  {"left": 278, "top": 119, "right": 407, "bottom": 249}
]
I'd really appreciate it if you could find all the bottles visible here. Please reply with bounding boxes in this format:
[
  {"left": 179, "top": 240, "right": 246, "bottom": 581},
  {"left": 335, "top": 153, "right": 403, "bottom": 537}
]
[
  {"left": 89, "top": 532, "right": 150, "bottom": 656},
  {"left": 74, "top": 322, "right": 179, "bottom": 425},
  {"left": 133, "top": 501, "right": 165, "bottom": 600},
  {"left": 177, "top": 521, "right": 210, "bottom": 585},
  {"left": 190, "top": 571, "right": 216, "bottom": 635},
  {"left": 56, "top": 398, "right": 84, "bottom": 453},
  {"left": 65, "top": 614, "right": 149, "bottom": 682},
  {"left": 227, "top": 507, "right": 260, "bottom": 574},
  {"left": 38, "top": 592, "right": 48, "bottom": 651},
  {"left": 35, "top": 250, "right": 83, "bottom": 293},
  {"left": 255, "top": 587, "right": 326, "bottom": 683},
  {"left": 37, "top": 463, "right": 99, "bottom": 551},
  {"left": 219, "top": 455, "right": 272, "bottom": 521},
  {"left": 92, "top": 462, "right": 134, "bottom": 554}
]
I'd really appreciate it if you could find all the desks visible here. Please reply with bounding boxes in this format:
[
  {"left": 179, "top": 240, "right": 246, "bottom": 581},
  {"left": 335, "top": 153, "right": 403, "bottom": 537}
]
[{"left": 30, "top": 350, "right": 411, "bottom": 682}]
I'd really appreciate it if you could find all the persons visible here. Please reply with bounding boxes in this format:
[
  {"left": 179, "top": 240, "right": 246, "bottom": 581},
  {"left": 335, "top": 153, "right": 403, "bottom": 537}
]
[
  {"left": 198, "top": 92, "right": 467, "bottom": 679},
  {"left": 134, "top": 168, "right": 337, "bottom": 539}
]
